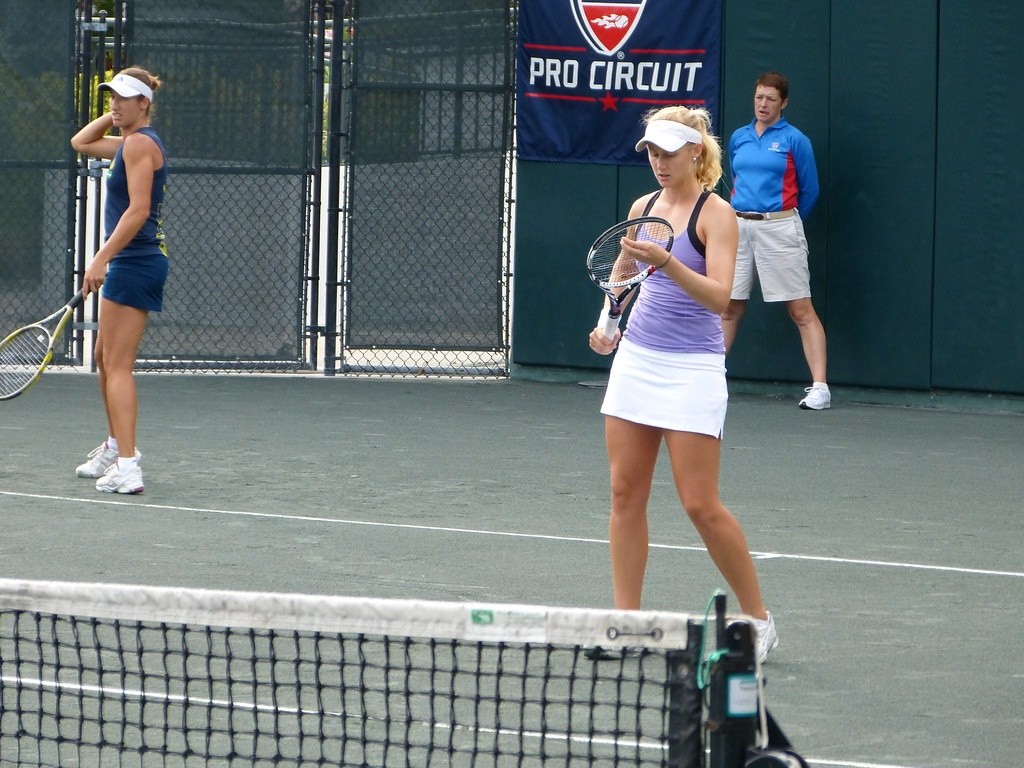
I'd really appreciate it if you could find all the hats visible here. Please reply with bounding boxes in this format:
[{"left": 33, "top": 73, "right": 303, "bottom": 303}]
[
  {"left": 96, "top": 74, "right": 153, "bottom": 104},
  {"left": 635, "top": 120, "right": 704, "bottom": 153}
]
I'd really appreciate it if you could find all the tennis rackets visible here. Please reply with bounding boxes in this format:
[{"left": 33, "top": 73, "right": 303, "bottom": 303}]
[
  {"left": 583, "top": 215, "right": 675, "bottom": 341},
  {"left": 0, "top": 285, "right": 92, "bottom": 403}
]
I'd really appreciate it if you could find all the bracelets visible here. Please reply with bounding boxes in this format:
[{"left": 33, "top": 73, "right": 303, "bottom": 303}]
[{"left": 655, "top": 252, "right": 672, "bottom": 270}]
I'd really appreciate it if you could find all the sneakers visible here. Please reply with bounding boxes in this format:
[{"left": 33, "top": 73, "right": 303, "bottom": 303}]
[
  {"left": 75, "top": 442, "right": 143, "bottom": 479},
  {"left": 746, "top": 612, "right": 780, "bottom": 665},
  {"left": 798, "top": 386, "right": 831, "bottom": 410},
  {"left": 96, "top": 461, "right": 145, "bottom": 494}
]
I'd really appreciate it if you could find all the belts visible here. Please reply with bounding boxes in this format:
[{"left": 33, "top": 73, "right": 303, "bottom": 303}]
[{"left": 735, "top": 208, "right": 798, "bottom": 220}]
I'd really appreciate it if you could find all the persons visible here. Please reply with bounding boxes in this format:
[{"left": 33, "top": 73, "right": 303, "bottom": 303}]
[
  {"left": 721, "top": 74, "right": 831, "bottom": 410},
  {"left": 589, "top": 105, "right": 779, "bottom": 664},
  {"left": 71, "top": 67, "right": 169, "bottom": 495}
]
